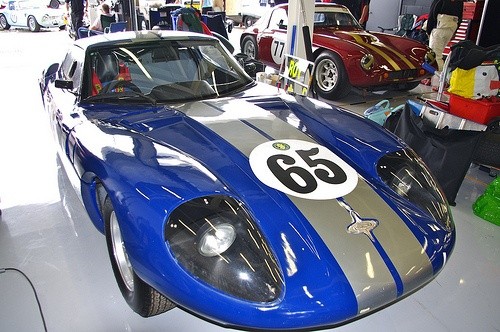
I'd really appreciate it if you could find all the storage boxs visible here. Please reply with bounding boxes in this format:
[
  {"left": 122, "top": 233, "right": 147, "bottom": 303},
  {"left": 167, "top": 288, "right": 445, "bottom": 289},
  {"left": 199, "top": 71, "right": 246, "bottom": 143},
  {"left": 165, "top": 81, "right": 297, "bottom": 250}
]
[{"left": 441, "top": 91, "right": 500, "bottom": 125}]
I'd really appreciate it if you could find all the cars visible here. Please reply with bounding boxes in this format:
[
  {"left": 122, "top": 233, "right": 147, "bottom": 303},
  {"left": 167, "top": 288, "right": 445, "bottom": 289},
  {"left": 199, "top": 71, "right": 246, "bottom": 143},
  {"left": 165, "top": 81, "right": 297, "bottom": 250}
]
[{"left": 0, "top": 1, "right": 68, "bottom": 32}]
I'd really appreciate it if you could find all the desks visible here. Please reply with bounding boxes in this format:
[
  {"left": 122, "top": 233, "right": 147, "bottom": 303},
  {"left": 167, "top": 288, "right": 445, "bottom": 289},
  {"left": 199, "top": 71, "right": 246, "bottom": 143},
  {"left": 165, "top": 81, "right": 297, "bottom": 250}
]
[{"left": 410, "top": 89, "right": 488, "bottom": 131}]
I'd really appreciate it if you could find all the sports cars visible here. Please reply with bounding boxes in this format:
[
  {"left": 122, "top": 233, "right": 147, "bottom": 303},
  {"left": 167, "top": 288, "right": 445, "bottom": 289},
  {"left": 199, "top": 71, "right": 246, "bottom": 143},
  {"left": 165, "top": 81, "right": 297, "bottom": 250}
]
[
  {"left": 240, "top": 2, "right": 441, "bottom": 99},
  {"left": 37, "top": 27, "right": 457, "bottom": 332}
]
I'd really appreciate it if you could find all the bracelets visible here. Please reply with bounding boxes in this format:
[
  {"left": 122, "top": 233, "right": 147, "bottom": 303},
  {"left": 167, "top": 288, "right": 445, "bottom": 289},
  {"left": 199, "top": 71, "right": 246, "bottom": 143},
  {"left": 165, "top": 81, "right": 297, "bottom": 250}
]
[{"left": 86, "top": 10, "right": 87, "bottom": 11}]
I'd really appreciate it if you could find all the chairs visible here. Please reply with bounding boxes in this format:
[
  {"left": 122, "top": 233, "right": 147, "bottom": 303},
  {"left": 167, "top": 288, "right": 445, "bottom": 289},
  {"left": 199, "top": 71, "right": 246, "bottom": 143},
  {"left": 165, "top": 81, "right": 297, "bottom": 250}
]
[
  {"left": 79, "top": 12, "right": 130, "bottom": 37},
  {"left": 95, "top": 53, "right": 121, "bottom": 82},
  {"left": 378, "top": 12, "right": 430, "bottom": 43},
  {"left": 323, "top": 18, "right": 338, "bottom": 24},
  {"left": 169, "top": 7, "right": 213, "bottom": 35}
]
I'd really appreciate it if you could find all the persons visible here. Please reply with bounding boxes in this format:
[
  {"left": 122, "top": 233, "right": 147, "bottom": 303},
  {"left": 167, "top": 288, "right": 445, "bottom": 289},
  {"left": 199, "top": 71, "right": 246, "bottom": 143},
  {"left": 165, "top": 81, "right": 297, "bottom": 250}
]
[
  {"left": 166, "top": 0, "right": 224, "bottom": 11},
  {"left": 315, "top": 0, "right": 371, "bottom": 28},
  {"left": 65, "top": 0, "right": 88, "bottom": 40},
  {"left": 419, "top": 0, "right": 464, "bottom": 90},
  {"left": 78, "top": 4, "right": 112, "bottom": 39}
]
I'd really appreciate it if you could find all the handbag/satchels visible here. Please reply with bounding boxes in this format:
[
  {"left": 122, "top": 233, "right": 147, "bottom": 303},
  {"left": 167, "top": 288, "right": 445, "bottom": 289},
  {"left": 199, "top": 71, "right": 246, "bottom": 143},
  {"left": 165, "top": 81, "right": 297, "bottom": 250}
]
[
  {"left": 472, "top": 173, "right": 500, "bottom": 226},
  {"left": 364, "top": 101, "right": 486, "bottom": 207}
]
